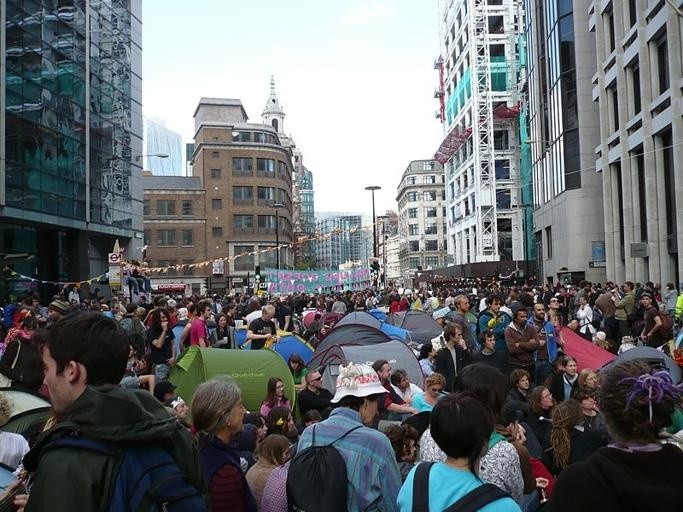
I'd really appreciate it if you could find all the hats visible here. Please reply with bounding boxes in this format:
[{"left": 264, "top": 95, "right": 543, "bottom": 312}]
[
  {"left": 329, "top": 362, "right": 390, "bottom": 405},
  {"left": 153, "top": 380, "right": 178, "bottom": 395},
  {"left": 47, "top": 300, "right": 68, "bottom": 314},
  {"left": 596, "top": 331, "right": 607, "bottom": 341},
  {"left": 641, "top": 293, "right": 651, "bottom": 299}
]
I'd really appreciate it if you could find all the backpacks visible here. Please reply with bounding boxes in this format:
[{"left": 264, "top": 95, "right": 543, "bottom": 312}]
[
  {"left": 657, "top": 309, "right": 674, "bottom": 340},
  {"left": 583, "top": 307, "right": 601, "bottom": 330},
  {"left": 33, "top": 431, "right": 207, "bottom": 511}
]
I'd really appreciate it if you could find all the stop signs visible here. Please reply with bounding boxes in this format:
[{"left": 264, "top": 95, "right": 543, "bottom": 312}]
[{"left": 109, "top": 253, "right": 119, "bottom": 263}]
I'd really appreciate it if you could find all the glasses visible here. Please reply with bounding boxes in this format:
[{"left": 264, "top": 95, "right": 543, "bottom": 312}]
[
  {"left": 430, "top": 385, "right": 445, "bottom": 393},
  {"left": 311, "top": 375, "right": 323, "bottom": 385}
]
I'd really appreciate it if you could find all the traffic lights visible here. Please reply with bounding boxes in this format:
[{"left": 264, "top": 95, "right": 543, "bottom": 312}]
[
  {"left": 202, "top": 277, "right": 209, "bottom": 289},
  {"left": 255, "top": 266, "right": 261, "bottom": 284},
  {"left": 224, "top": 276, "right": 230, "bottom": 289},
  {"left": 370, "top": 260, "right": 381, "bottom": 280}
]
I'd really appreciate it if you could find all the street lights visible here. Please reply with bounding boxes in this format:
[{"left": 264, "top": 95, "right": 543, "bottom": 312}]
[
  {"left": 363, "top": 185, "right": 382, "bottom": 289},
  {"left": 511, "top": 204, "right": 533, "bottom": 286},
  {"left": 375, "top": 215, "right": 389, "bottom": 289},
  {"left": 269, "top": 203, "right": 287, "bottom": 272}
]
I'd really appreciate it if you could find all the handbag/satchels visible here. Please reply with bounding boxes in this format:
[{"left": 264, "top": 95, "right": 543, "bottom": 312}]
[
  {"left": 285, "top": 424, "right": 362, "bottom": 511},
  {"left": 626, "top": 310, "right": 640, "bottom": 322}
]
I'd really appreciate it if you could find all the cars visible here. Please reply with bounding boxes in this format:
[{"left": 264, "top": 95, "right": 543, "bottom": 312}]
[{"left": 497, "top": 268, "right": 537, "bottom": 285}]
